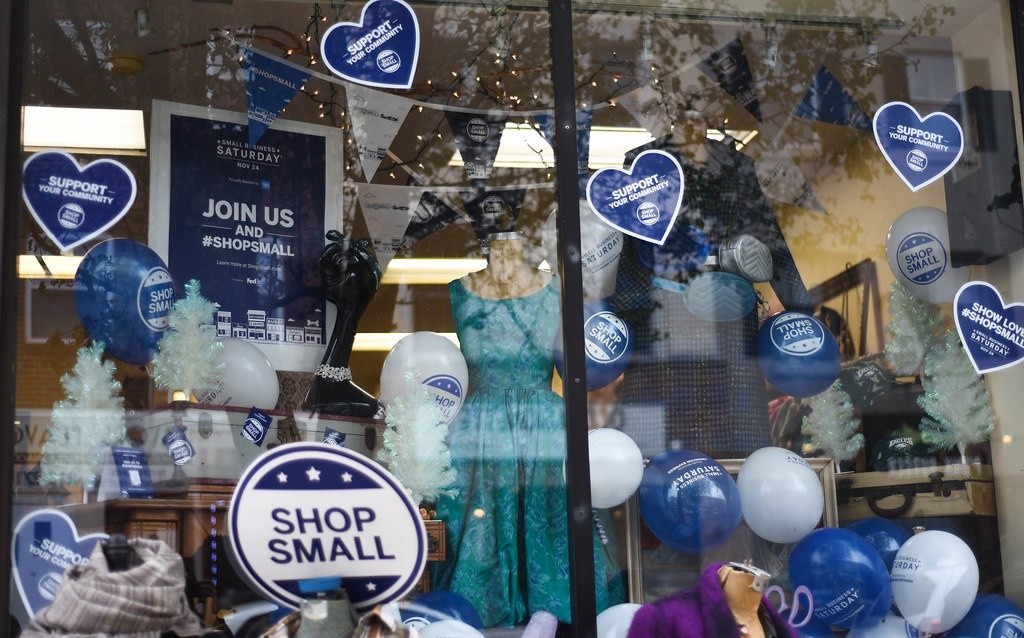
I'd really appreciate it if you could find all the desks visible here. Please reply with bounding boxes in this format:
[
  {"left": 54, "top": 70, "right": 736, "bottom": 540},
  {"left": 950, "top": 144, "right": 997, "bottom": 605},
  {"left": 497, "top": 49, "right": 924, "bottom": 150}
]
[{"left": 50, "top": 483, "right": 445, "bottom": 638}]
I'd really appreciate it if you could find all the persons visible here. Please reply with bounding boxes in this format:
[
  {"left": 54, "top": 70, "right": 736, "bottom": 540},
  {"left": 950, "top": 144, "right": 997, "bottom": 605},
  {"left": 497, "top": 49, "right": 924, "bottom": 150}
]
[
  {"left": 613, "top": 104, "right": 814, "bottom": 459},
  {"left": 445, "top": 225, "right": 629, "bottom": 626},
  {"left": 20, "top": 528, "right": 808, "bottom": 637}
]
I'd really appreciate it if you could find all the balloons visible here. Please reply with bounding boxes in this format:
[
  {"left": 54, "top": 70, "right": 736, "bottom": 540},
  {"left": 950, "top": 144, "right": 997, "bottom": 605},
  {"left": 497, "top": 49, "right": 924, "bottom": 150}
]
[
  {"left": 637, "top": 451, "right": 740, "bottom": 552},
  {"left": 541, "top": 200, "right": 625, "bottom": 298},
  {"left": 950, "top": 590, "right": 1024, "bottom": 638},
  {"left": 73, "top": 237, "right": 183, "bottom": 367},
  {"left": 789, "top": 527, "right": 895, "bottom": 624},
  {"left": 883, "top": 205, "right": 972, "bottom": 307},
  {"left": 376, "top": 337, "right": 475, "bottom": 427},
  {"left": 735, "top": 446, "right": 828, "bottom": 543},
  {"left": 778, "top": 609, "right": 834, "bottom": 637},
  {"left": 190, "top": 335, "right": 283, "bottom": 414},
  {"left": 548, "top": 300, "right": 633, "bottom": 389},
  {"left": 840, "top": 616, "right": 927, "bottom": 638},
  {"left": 894, "top": 530, "right": 980, "bottom": 633},
  {"left": 851, "top": 515, "right": 914, "bottom": 575},
  {"left": 560, "top": 430, "right": 642, "bottom": 510},
  {"left": 754, "top": 307, "right": 840, "bottom": 399}
]
